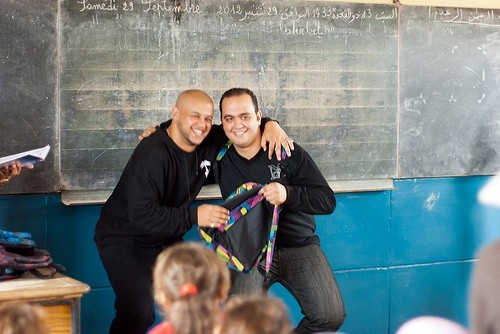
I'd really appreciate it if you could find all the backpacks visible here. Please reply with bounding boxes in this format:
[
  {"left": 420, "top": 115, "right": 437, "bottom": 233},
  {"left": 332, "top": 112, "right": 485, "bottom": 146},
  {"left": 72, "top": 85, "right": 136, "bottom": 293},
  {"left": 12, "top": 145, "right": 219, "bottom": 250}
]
[{"left": 196, "top": 181, "right": 280, "bottom": 282}]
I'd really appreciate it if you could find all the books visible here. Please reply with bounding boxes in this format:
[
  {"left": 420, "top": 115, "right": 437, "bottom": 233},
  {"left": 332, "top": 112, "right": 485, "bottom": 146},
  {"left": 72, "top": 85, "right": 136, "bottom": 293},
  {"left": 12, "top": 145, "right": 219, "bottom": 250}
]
[{"left": 0, "top": 144, "right": 50, "bottom": 169}]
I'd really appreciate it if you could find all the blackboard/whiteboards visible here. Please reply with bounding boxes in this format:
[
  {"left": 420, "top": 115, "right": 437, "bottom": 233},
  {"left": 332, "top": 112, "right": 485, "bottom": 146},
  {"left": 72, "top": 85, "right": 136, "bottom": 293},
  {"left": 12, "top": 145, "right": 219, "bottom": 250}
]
[{"left": 0, "top": 0, "right": 500, "bottom": 207}]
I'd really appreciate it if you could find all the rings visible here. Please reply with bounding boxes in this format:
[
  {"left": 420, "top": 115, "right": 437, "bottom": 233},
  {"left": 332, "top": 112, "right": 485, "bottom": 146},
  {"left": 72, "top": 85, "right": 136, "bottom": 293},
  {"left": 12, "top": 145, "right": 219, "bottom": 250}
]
[{"left": 210, "top": 222, "right": 215, "bottom": 229}]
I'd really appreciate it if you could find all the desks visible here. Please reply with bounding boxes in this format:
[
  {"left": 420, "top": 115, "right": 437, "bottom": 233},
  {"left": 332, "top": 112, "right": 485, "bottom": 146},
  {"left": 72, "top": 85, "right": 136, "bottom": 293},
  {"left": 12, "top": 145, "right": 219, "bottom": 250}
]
[{"left": 0, "top": 267, "right": 91, "bottom": 334}]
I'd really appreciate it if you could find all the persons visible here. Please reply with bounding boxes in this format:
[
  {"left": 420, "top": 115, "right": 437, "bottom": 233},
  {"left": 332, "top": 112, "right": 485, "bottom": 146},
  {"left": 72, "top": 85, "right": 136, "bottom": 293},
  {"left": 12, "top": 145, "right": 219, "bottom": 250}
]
[
  {"left": 148, "top": 241, "right": 292, "bottom": 334},
  {"left": 0, "top": 161, "right": 34, "bottom": 185},
  {"left": 138, "top": 88, "right": 346, "bottom": 334},
  {"left": 94, "top": 89, "right": 295, "bottom": 334}
]
